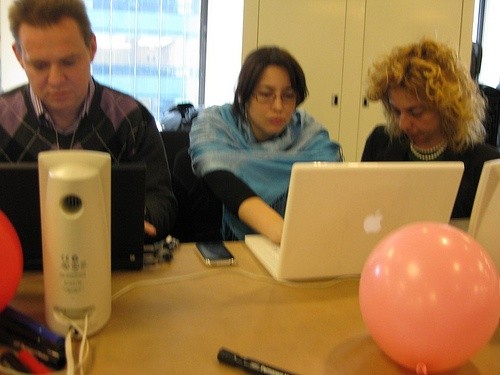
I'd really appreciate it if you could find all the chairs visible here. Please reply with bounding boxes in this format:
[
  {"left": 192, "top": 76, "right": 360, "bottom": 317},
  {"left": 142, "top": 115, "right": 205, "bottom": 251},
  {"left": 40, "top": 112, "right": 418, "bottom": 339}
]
[{"left": 162, "top": 130, "right": 214, "bottom": 240}]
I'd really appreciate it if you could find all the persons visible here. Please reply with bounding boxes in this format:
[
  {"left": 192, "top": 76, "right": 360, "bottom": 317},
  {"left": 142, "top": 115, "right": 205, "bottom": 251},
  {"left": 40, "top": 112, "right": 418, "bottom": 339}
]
[
  {"left": 0, "top": 0, "right": 172, "bottom": 242},
  {"left": 187, "top": 45, "right": 340, "bottom": 238},
  {"left": 359, "top": 220, "right": 500, "bottom": 375},
  {"left": 361, "top": 37, "right": 500, "bottom": 219}
]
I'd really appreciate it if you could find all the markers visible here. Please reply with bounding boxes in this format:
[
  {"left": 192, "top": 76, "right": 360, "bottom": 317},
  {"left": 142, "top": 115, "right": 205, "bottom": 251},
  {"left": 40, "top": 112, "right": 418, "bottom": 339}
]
[{"left": 217, "top": 348, "right": 297, "bottom": 375}]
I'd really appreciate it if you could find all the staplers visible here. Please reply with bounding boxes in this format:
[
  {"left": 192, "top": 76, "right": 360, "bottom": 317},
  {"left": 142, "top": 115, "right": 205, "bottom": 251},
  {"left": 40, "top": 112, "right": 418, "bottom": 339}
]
[{"left": 0, "top": 307, "right": 67, "bottom": 375}]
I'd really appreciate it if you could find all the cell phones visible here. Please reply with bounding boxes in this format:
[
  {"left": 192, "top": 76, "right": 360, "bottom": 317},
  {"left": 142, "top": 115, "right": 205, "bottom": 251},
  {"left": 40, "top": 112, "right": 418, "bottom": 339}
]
[{"left": 195, "top": 241, "right": 234, "bottom": 266}]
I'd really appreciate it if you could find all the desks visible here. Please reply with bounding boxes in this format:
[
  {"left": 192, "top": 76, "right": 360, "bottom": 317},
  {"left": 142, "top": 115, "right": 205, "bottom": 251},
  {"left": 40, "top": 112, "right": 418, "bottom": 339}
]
[{"left": 5, "top": 219, "right": 500, "bottom": 375}]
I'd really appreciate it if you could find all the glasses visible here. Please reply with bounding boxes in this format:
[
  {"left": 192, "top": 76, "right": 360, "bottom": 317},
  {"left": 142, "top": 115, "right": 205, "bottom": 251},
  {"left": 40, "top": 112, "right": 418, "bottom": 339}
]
[{"left": 251, "top": 90, "right": 297, "bottom": 105}]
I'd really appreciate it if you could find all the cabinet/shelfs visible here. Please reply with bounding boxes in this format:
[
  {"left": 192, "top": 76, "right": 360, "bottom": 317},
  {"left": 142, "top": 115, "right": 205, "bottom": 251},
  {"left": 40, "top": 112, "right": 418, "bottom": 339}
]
[{"left": 243, "top": 0, "right": 476, "bottom": 162}]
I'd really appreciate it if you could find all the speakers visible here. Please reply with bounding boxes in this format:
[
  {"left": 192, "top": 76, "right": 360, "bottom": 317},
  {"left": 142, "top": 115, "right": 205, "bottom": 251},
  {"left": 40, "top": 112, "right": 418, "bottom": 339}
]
[{"left": 38, "top": 150, "right": 111, "bottom": 338}]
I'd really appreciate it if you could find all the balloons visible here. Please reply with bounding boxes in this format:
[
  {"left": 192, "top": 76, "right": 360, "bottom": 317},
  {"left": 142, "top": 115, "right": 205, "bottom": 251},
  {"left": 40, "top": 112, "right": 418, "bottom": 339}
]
[{"left": 0, "top": 210, "right": 23, "bottom": 312}]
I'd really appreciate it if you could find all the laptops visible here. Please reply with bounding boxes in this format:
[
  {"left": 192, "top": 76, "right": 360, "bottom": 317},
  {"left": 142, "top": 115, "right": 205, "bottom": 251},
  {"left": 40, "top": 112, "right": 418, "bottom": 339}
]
[
  {"left": 1, "top": 162, "right": 145, "bottom": 271},
  {"left": 469, "top": 159, "right": 500, "bottom": 277},
  {"left": 244, "top": 162, "right": 465, "bottom": 282}
]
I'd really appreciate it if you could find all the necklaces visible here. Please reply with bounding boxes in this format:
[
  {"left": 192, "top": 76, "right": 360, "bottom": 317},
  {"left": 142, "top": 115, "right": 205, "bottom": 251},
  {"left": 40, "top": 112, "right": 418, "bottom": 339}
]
[{"left": 409, "top": 140, "right": 446, "bottom": 160}]
